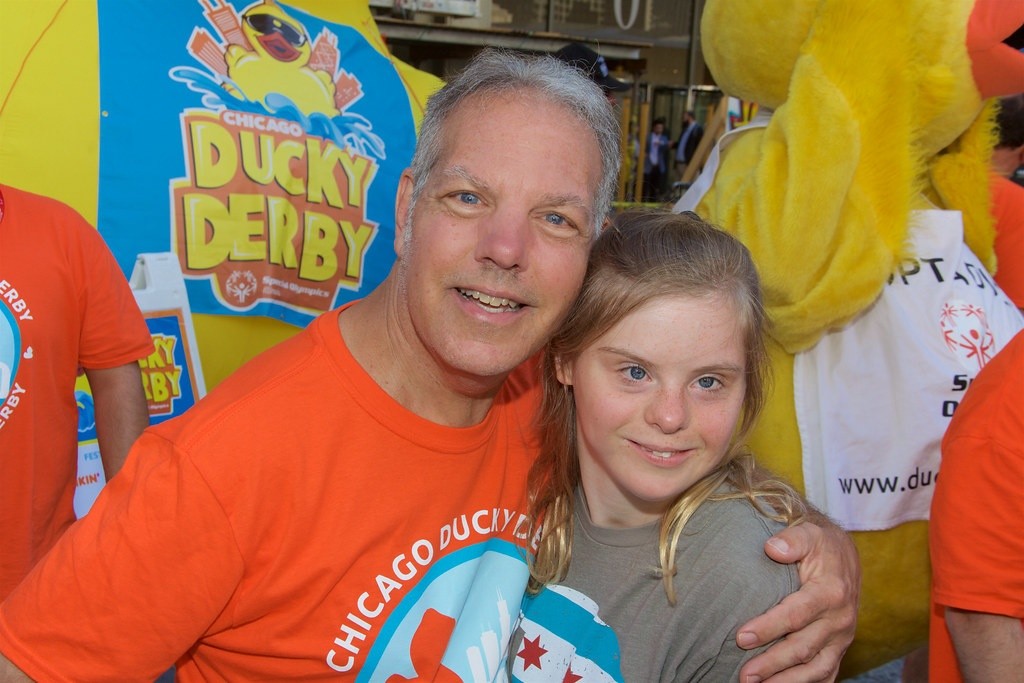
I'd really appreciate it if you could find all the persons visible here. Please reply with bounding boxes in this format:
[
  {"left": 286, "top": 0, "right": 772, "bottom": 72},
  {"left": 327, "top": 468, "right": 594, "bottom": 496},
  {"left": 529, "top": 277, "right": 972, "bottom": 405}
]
[
  {"left": 495, "top": 210, "right": 803, "bottom": 683},
  {"left": 0, "top": 46, "right": 860, "bottom": 683},
  {"left": 990, "top": 97, "right": 1024, "bottom": 317},
  {"left": 626, "top": 109, "right": 703, "bottom": 204},
  {"left": 924, "top": 327, "right": 1024, "bottom": 682},
  {"left": 0, "top": 185, "right": 156, "bottom": 603}
]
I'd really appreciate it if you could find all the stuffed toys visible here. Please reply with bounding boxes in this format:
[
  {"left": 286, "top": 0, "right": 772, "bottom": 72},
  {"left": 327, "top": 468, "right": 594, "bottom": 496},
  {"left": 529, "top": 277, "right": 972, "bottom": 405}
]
[{"left": 680, "top": 1, "right": 1024, "bottom": 674}]
